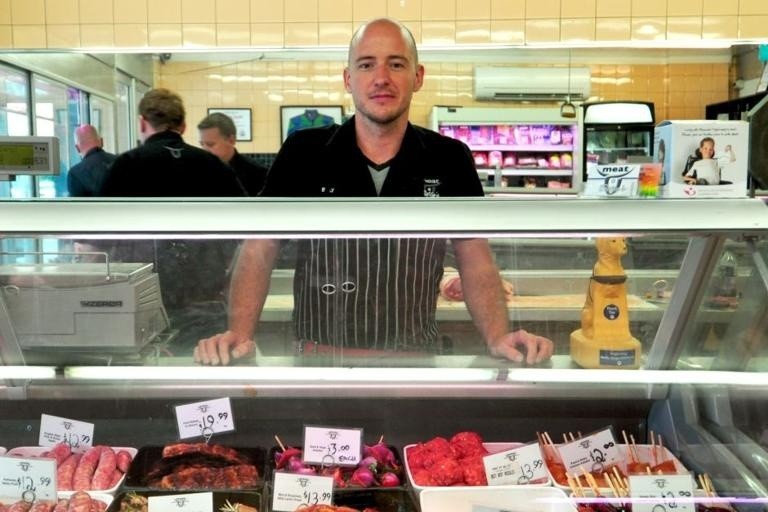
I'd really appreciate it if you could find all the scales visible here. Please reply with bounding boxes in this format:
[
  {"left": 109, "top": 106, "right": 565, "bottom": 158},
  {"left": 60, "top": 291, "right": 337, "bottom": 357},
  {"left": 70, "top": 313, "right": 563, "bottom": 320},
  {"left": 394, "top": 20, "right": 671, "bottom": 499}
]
[{"left": 0, "top": 136, "right": 171, "bottom": 352}]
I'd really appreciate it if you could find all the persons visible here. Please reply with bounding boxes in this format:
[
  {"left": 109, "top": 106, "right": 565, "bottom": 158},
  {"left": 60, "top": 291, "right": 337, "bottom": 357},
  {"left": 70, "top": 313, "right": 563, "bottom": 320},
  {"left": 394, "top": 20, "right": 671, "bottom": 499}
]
[
  {"left": 653, "top": 140, "right": 667, "bottom": 188},
  {"left": 195, "top": 111, "right": 267, "bottom": 198},
  {"left": 184, "top": 14, "right": 554, "bottom": 368},
  {"left": 98, "top": 87, "right": 245, "bottom": 341},
  {"left": 65, "top": 121, "right": 118, "bottom": 198},
  {"left": 285, "top": 108, "right": 334, "bottom": 135},
  {"left": 682, "top": 138, "right": 735, "bottom": 190}
]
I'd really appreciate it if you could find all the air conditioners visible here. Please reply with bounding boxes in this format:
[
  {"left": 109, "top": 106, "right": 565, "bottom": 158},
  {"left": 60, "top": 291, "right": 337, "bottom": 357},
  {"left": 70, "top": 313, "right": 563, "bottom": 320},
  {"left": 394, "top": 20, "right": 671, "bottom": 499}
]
[{"left": 471, "top": 63, "right": 593, "bottom": 102}]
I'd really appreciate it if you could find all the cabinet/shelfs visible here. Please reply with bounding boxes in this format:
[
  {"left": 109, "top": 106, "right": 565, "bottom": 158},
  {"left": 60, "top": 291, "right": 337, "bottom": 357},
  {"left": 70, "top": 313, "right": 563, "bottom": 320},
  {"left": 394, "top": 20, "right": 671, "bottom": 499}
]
[
  {"left": 424, "top": 104, "right": 584, "bottom": 193},
  {"left": 0, "top": 193, "right": 764, "bottom": 512}
]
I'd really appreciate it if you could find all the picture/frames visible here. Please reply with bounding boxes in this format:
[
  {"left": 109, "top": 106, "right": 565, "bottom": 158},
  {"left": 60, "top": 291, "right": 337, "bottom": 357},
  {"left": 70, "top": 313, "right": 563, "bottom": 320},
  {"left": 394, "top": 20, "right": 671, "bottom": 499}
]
[
  {"left": 206, "top": 106, "right": 253, "bottom": 143},
  {"left": 278, "top": 102, "right": 345, "bottom": 149}
]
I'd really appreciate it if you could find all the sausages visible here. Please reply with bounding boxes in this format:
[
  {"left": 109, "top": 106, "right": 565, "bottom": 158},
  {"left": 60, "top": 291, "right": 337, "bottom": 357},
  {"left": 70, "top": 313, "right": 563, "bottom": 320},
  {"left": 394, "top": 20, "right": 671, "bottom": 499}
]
[
  {"left": 10, "top": 441, "right": 130, "bottom": 490},
  {"left": 0, "top": 493, "right": 104, "bottom": 512}
]
